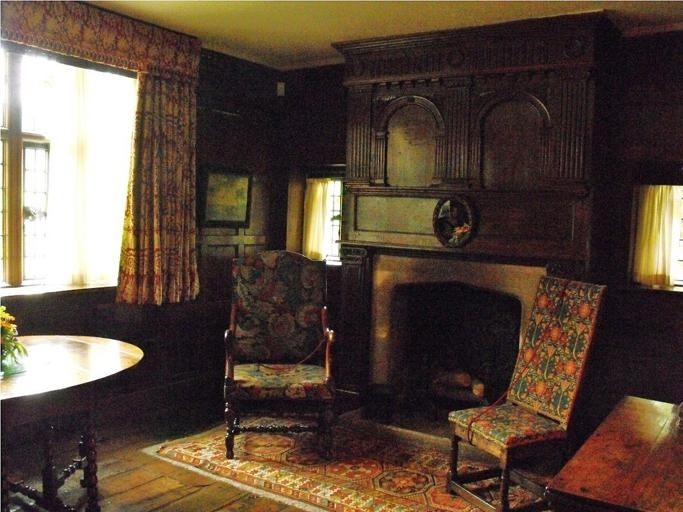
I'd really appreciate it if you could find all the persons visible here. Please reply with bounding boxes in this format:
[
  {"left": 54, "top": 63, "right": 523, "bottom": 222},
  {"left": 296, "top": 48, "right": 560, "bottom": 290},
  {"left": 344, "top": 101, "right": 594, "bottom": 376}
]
[{"left": 439, "top": 205, "right": 469, "bottom": 239}]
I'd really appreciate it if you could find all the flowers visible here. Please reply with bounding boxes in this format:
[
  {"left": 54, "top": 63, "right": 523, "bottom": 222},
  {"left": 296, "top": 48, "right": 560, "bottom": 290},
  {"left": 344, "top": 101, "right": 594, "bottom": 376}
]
[
  {"left": 446, "top": 220, "right": 470, "bottom": 239},
  {"left": 1, "top": 301, "right": 29, "bottom": 373}
]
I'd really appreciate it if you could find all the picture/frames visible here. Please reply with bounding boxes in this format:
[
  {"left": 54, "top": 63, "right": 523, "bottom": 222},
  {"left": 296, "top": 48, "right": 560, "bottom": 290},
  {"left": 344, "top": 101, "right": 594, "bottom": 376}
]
[{"left": 196, "top": 163, "right": 255, "bottom": 229}]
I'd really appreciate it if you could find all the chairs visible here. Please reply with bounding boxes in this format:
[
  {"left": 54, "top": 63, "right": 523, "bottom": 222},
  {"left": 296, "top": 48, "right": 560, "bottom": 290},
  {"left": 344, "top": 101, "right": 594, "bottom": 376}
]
[
  {"left": 221, "top": 251, "right": 336, "bottom": 457},
  {"left": 443, "top": 272, "right": 611, "bottom": 507}
]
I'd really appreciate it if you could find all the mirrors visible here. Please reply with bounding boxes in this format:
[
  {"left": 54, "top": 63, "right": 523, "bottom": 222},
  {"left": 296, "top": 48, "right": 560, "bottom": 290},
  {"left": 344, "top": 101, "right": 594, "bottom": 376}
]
[{"left": 429, "top": 193, "right": 476, "bottom": 249}]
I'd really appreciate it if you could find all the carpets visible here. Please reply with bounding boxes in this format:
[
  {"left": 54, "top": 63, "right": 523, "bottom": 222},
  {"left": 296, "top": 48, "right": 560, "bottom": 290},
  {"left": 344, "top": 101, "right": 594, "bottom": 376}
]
[{"left": 144, "top": 405, "right": 560, "bottom": 512}]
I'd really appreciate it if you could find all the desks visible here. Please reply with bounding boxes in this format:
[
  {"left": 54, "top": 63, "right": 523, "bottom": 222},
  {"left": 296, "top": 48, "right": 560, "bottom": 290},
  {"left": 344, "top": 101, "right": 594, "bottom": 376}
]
[
  {"left": 542, "top": 391, "right": 683, "bottom": 511},
  {"left": 1, "top": 334, "right": 146, "bottom": 509}
]
